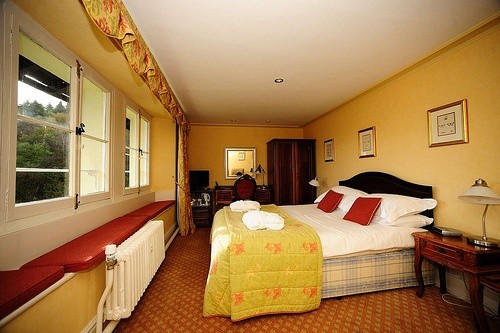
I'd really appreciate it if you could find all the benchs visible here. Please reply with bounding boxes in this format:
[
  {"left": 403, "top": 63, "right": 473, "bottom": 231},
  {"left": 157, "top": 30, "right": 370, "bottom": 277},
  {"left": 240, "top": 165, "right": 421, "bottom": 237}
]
[{"left": 0, "top": 199, "right": 178, "bottom": 333}]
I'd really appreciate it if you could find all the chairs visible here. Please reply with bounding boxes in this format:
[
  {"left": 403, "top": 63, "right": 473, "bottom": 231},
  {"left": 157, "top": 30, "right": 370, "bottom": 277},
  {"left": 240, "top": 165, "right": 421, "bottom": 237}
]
[{"left": 233, "top": 174, "right": 257, "bottom": 202}]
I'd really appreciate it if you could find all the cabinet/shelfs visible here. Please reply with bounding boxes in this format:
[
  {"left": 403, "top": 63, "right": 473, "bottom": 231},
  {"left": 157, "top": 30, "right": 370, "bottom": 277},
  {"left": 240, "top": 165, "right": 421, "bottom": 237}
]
[
  {"left": 213, "top": 186, "right": 269, "bottom": 215},
  {"left": 266, "top": 138, "right": 316, "bottom": 206},
  {"left": 192, "top": 205, "right": 213, "bottom": 229}
]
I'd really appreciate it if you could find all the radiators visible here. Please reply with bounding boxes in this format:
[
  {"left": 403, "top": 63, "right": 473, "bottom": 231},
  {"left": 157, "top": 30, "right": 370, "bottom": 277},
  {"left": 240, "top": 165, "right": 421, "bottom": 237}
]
[{"left": 105, "top": 220, "right": 166, "bottom": 320}]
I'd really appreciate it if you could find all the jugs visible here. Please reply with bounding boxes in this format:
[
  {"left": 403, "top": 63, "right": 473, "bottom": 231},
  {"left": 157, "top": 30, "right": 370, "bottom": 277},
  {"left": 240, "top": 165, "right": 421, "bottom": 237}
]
[{"left": 201, "top": 193, "right": 210, "bottom": 206}]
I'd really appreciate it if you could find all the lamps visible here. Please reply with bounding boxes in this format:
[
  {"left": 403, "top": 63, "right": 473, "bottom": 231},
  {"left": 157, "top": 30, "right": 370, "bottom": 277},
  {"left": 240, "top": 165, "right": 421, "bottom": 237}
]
[
  {"left": 309, "top": 177, "right": 320, "bottom": 202},
  {"left": 458, "top": 178, "right": 500, "bottom": 246}
]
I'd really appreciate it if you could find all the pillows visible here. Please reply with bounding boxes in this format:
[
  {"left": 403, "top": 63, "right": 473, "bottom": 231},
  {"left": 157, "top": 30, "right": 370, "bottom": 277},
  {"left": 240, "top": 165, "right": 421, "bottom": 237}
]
[{"left": 314, "top": 185, "right": 437, "bottom": 228}]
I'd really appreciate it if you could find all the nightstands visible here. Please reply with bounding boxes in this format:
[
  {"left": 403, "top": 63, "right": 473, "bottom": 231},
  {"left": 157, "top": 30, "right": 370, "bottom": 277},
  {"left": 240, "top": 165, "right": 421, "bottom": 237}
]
[{"left": 411, "top": 231, "right": 500, "bottom": 333}]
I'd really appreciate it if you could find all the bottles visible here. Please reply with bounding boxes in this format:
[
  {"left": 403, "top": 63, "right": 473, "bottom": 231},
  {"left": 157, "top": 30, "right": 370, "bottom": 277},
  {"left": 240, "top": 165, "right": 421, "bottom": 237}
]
[{"left": 197, "top": 198, "right": 202, "bottom": 206}]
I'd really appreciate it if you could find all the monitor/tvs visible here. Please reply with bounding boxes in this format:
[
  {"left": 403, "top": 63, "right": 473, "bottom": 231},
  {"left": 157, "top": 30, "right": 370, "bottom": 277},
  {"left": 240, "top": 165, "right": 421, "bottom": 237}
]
[{"left": 189, "top": 171, "right": 209, "bottom": 191}]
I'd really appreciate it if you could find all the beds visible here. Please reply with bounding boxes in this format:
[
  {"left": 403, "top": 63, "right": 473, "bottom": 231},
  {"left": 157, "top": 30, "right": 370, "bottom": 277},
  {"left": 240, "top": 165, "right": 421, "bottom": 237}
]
[{"left": 202, "top": 171, "right": 437, "bottom": 322}]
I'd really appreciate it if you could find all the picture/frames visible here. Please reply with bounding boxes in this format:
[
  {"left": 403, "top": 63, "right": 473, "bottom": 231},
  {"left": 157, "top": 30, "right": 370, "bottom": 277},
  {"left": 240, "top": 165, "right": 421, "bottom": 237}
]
[
  {"left": 324, "top": 138, "right": 335, "bottom": 162},
  {"left": 358, "top": 126, "right": 376, "bottom": 158},
  {"left": 427, "top": 99, "right": 470, "bottom": 148}
]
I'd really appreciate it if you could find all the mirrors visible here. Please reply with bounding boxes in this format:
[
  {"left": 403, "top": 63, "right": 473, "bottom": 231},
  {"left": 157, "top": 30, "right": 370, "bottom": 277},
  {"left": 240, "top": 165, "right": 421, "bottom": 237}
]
[{"left": 225, "top": 147, "right": 257, "bottom": 180}]
